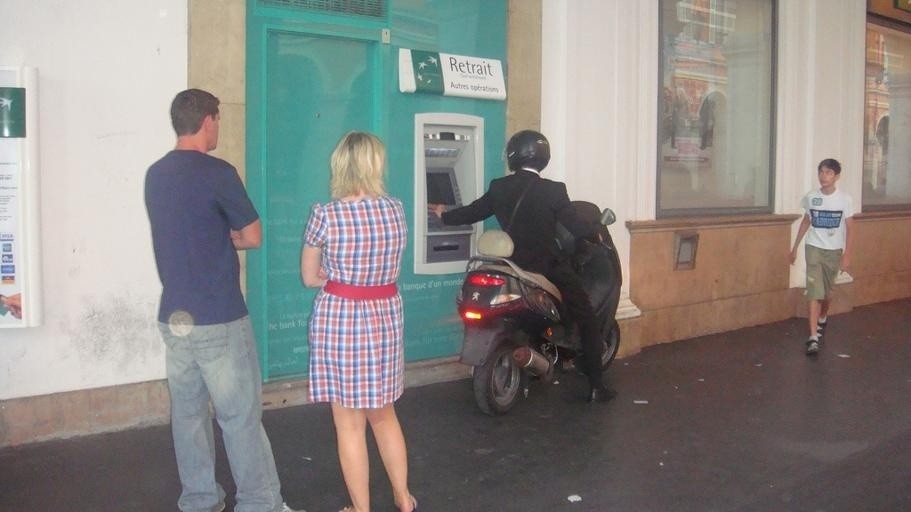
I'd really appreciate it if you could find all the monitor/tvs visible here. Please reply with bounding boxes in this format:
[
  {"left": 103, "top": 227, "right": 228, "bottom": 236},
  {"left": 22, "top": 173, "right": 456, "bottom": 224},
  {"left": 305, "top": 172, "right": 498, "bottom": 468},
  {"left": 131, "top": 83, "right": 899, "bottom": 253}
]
[{"left": 427, "top": 173, "right": 456, "bottom": 206}]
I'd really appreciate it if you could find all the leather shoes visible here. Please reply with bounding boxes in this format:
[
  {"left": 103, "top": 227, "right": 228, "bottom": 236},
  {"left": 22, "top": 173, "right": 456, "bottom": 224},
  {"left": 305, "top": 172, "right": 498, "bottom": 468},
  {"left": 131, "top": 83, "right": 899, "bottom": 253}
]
[{"left": 590, "top": 388, "right": 617, "bottom": 402}]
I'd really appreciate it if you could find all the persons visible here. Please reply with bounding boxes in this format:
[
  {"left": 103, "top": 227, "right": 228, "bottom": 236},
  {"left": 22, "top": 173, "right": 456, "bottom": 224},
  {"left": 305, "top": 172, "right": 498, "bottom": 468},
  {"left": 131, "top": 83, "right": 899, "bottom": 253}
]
[
  {"left": 145, "top": 89, "right": 306, "bottom": 512},
  {"left": 300, "top": 130, "right": 420, "bottom": 512},
  {"left": 428, "top": 130, "right": 619, "bottom": 404},
  {"left": 790, "top": 159, "right": 855, "bottom": 355}
]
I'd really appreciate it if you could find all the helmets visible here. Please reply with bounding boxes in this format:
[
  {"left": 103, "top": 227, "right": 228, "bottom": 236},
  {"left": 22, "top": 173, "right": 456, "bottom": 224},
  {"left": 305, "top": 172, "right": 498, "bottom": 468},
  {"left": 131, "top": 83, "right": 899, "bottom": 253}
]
[{"left": 501, "top": 130, "right": 550, "bottom": 171}]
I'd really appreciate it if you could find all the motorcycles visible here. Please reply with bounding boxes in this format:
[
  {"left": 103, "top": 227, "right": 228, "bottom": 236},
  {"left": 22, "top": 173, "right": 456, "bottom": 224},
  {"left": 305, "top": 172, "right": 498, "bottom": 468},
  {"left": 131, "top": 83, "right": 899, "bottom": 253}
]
[{"left": 456, "top": 199, "right": 622, "bottom": 417}]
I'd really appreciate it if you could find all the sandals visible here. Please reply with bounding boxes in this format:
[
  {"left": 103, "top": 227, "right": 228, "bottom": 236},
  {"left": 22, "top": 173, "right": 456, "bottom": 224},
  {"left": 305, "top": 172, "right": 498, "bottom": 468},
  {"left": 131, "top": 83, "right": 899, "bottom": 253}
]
[
  {"left": 806, "top": 340, "right": 818, "bottom": 354},
  {"left": 817, "top": 319, "right": 826, "bottom": 338}
]
